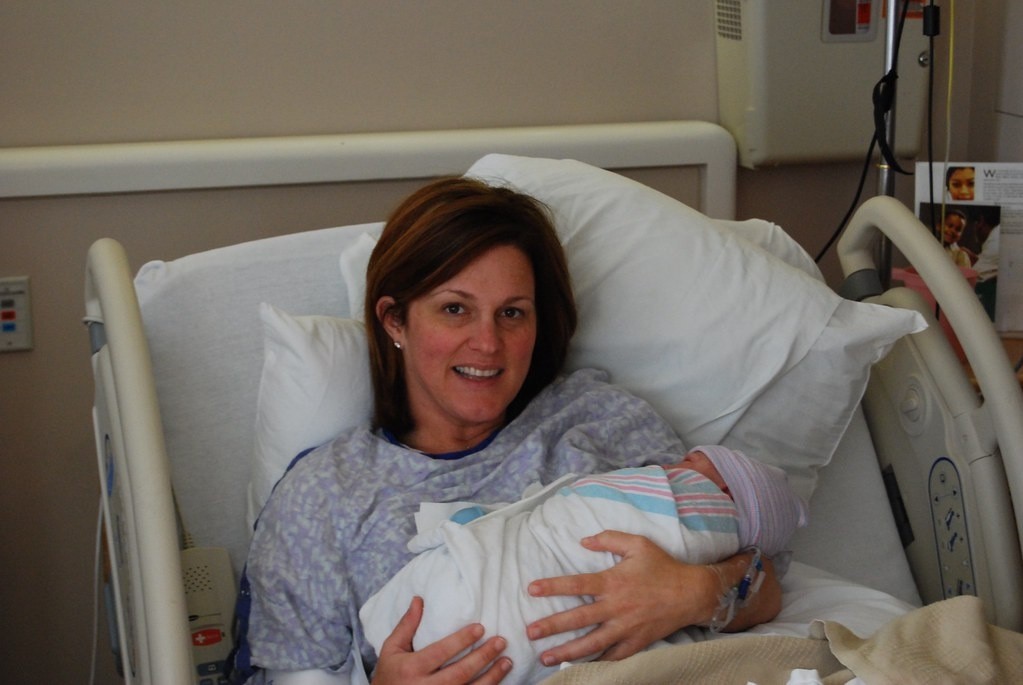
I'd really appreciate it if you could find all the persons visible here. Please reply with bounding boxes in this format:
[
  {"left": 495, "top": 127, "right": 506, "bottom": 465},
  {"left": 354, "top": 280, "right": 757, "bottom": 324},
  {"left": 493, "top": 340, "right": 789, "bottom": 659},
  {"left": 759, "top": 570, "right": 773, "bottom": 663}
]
[
  {"left": 243, "top": 177, "right": 781, "bottom": 685},
  {"left": 946, "top": 166, "right": 975, "bottom": 201},
  {"left": 962, "top": 210, "right": 1000, "bottom": 323},
  {"left": 936, "top": 210, "right": 971, "bottom": 272},
  {"left": 360, "top": 444, "right": 809, "bottom": 685}
]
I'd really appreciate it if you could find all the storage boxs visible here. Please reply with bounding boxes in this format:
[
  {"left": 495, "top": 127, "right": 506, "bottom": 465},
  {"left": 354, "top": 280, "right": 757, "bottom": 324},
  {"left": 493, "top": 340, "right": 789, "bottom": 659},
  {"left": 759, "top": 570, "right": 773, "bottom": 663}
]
[{"left": 890, "top": 265, "right": 979, "bottom": 362}]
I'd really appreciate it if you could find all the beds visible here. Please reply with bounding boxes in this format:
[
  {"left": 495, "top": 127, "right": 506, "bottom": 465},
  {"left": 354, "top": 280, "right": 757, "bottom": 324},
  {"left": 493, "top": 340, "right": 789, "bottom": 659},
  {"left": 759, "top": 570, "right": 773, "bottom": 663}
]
[{"left": 82, "top": 192, "right": 1023, "bottom": 685}]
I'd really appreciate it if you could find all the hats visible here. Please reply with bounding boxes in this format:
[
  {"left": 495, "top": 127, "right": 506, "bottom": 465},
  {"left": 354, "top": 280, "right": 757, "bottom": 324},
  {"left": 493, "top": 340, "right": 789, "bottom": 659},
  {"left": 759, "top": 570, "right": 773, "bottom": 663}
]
[{"left": 689, "top": 444, "right": 808, "bottom": 555}]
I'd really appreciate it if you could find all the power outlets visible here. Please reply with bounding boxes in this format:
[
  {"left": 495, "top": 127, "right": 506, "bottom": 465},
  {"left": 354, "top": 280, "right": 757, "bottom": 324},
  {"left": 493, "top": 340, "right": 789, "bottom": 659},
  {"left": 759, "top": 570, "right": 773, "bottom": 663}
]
[{"left": 0, "top": 276, "right": 35, "bottom": 352}]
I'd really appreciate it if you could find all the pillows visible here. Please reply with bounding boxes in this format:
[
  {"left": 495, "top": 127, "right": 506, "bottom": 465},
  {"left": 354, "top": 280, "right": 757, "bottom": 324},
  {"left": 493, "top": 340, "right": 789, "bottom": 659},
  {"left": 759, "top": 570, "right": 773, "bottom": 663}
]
[
  {"left": 342, "top": 151, "right": 927, "bottom": 506},
  {"left": 249, "top": 300, "right": 375, "bottom": 530}
]
